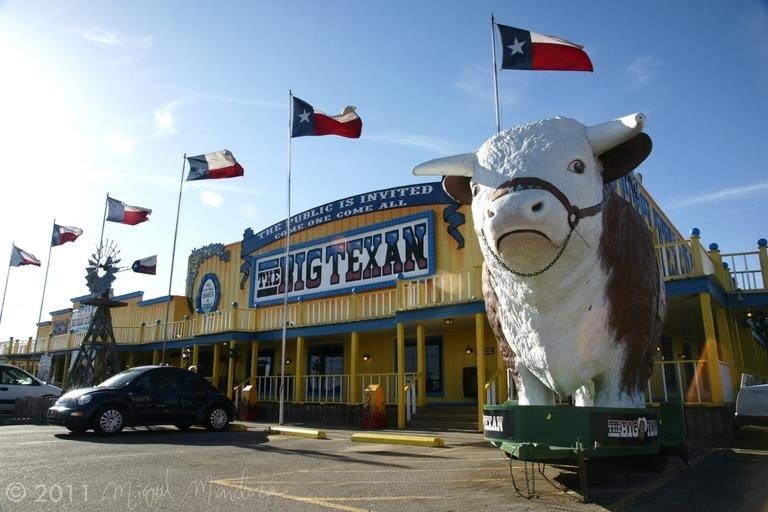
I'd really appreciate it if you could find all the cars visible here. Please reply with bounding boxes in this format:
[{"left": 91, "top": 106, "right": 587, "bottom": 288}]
[
  {"left": 0, "top": 364, "right": 62, "bottom": 414},
  {"left": 46, "top": 364, "right": 237, "bottom": 437}
]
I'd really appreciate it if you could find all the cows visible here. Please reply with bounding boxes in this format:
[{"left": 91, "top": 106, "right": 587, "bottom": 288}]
[{"left": 412, "top": 113, "right": 664, "bottom": 408}]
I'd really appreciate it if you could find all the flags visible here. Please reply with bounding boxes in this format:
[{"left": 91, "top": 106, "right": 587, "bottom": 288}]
[
  {"left": 10, "top": 245, "right": 40, "bottom": 266},
  {"left": 51, "top": 224, "right": 83, "bottom": 246},
  {"left": 184, "top": 149, "right": 244, "bottom": 181},
  {"left": 291, "top": 96, "right": 362, "bottom": 138},
  {"left": 495, "top": 22, "right": 592, "bottom": 71},
  {"left": 106, "top": 197, "right": 152, "bottom": 225}
]
